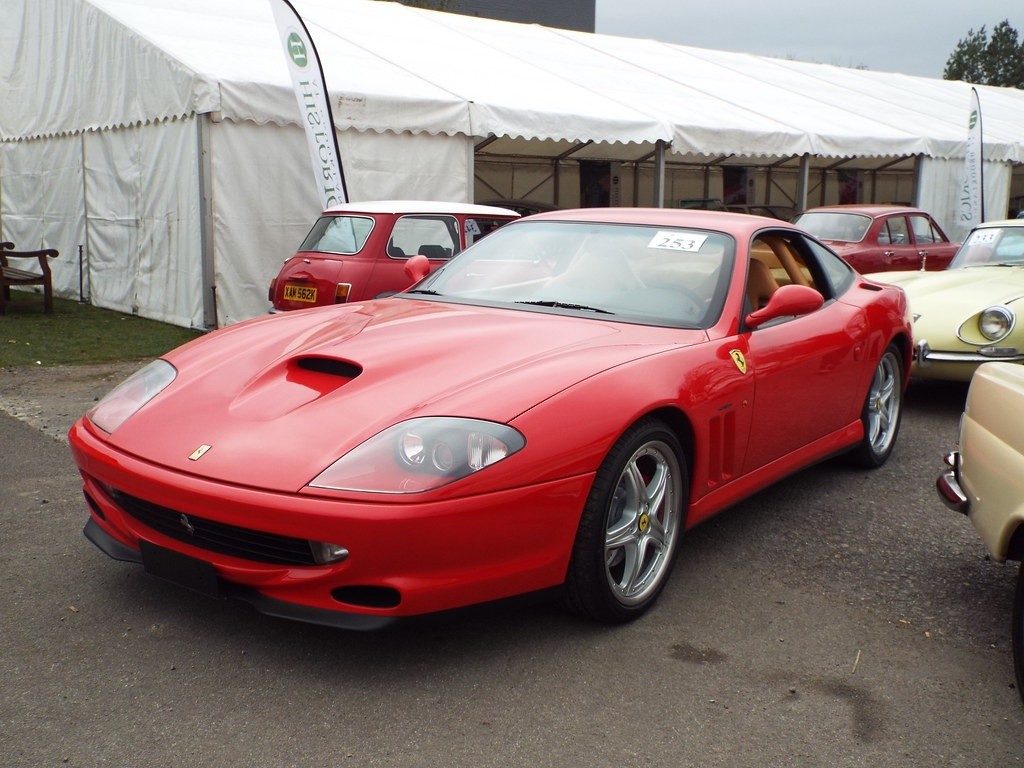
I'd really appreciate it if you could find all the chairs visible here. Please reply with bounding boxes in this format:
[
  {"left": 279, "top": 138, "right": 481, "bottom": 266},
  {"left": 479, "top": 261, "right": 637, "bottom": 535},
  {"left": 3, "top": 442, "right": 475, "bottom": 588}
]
[
  {"left": 388, "top": 237, "right": 406, "bottom": 257},
  {"left": 700, "top": 256, "right": 780, "bottom": 310},
  {"left": 418, "top": 245, "right": 446, "bottom": 258}
]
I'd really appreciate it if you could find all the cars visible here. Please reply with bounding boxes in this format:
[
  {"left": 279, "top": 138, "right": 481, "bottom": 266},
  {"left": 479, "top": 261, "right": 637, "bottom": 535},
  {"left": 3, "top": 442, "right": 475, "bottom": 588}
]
[
  {"left": 268, "top": 202, "right": 524, "bottom": 326},
  {"left": 854, "top": 219, "right": 1024, "bottom": 394},
  {"left": 933, "top": 363, "right": 1023, "bottom": 717},
  {"left": 788, "top": 204, "right": 1000, "bottom": 281}
]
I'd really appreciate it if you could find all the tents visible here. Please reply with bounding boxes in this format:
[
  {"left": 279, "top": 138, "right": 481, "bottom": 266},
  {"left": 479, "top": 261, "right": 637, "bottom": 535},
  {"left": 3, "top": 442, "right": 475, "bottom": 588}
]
[{"left": 0, "top": 1, "right": 1024, "bottom": 351}]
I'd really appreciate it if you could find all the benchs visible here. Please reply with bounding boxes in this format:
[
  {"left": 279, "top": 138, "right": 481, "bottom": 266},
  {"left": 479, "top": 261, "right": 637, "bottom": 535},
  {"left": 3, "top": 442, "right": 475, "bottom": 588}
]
[{"left": 0, "top": 241, "right": 60, "bottom": 316}]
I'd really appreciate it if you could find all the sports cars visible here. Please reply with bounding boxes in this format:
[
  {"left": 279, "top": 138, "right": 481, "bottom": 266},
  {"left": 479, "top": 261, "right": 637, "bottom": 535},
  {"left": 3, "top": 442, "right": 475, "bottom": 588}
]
[{"left": 64, "top": 205, "right": 914, "bottom": 633}]
